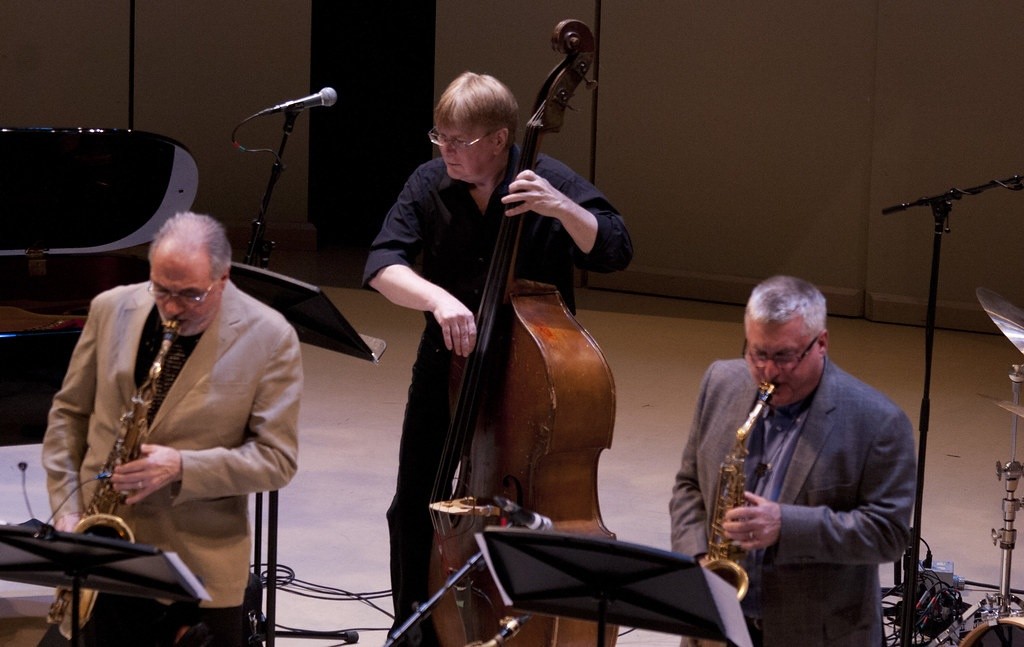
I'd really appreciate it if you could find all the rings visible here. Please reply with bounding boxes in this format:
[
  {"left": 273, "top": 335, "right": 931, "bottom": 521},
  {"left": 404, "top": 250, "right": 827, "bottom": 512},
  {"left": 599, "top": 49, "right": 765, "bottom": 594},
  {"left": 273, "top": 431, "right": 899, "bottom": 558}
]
[
  {"left": 135, "top": 482, "right": 144, "bottom": 492},
  {"left": 748, "top": 531, "right": 755, "bottom": 540}
]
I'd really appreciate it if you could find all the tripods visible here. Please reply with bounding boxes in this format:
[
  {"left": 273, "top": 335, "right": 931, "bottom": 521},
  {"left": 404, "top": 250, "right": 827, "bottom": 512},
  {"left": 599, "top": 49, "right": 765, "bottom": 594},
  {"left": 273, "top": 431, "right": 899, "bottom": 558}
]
[{"left": 924, "top": 364, "right": 1024, "bottom": 647}]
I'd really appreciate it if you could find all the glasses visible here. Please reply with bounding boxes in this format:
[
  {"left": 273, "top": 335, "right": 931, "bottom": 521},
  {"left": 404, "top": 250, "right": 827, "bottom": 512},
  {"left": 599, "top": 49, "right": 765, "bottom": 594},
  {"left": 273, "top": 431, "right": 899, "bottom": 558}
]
[
  {"left": 146, "top": 280, "right": 217, "bottom": 305},
  {"left": 739, "top": 334, "right": 821, "bottom": 373},
  {"left": 427, "top": 123, "right": 492, "bottom": 153}
]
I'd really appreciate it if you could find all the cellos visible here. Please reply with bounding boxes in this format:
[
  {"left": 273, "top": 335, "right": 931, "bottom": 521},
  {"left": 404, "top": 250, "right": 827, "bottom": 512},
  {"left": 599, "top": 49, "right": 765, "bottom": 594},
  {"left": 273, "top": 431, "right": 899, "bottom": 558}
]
[{"left": 428, "top": 16, "right": 621, "bottom": 647}]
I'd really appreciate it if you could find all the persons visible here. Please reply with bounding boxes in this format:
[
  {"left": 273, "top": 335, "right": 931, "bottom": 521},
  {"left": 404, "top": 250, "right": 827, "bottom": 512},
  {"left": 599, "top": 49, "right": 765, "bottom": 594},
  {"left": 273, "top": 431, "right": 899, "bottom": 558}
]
[
  {"left": 41, "top": 211, "right": 304, "bottom": 647},
  {"left": 668, "top": 276, "right": 917, "bottom": 647},
  {"left": 366, "top": 72, "right": 637, "bottom": 647}
]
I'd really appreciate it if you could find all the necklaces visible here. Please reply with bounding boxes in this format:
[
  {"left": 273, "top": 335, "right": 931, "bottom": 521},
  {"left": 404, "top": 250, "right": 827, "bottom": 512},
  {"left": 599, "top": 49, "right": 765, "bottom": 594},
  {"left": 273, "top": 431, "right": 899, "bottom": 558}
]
[{"left": 760, "top": 416, "right": 798, "bottom": 470}]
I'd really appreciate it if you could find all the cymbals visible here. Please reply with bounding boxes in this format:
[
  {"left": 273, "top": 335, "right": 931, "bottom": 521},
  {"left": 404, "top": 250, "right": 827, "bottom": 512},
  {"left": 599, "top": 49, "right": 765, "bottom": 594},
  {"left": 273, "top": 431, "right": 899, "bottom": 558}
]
[
  {"left": 974, "top": 284, "right": 1023, "bottom": 352},
  {"left": 973, "top": 390, "right": 1024, "bottom": 422}
]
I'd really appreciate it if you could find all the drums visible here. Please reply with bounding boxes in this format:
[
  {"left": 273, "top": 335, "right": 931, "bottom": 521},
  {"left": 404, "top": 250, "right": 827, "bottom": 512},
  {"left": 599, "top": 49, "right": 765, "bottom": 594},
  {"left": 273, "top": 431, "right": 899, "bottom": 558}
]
[{"left": 958, "top": 613, "right": 1024, "bottom": 647}]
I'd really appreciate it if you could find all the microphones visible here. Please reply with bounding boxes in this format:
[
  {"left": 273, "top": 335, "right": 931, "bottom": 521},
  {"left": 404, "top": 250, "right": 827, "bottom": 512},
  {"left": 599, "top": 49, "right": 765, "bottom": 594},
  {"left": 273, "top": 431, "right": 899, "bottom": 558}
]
[
  {"left": 491, "top": 495, "right": 552, "bottom": 531},
  {"left": 256, "top": 87, "right": 337, "bottom": 118}
]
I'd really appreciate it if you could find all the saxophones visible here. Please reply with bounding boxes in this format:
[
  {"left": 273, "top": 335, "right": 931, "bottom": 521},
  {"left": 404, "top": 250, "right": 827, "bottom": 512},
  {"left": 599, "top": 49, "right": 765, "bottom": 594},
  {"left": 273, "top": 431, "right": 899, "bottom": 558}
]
[
  {"left": 45, "top": 317, "right": 180, "bottom": 646},
  {"left": 678, "top": 381, "right": 778, "bottom": 647}
]
